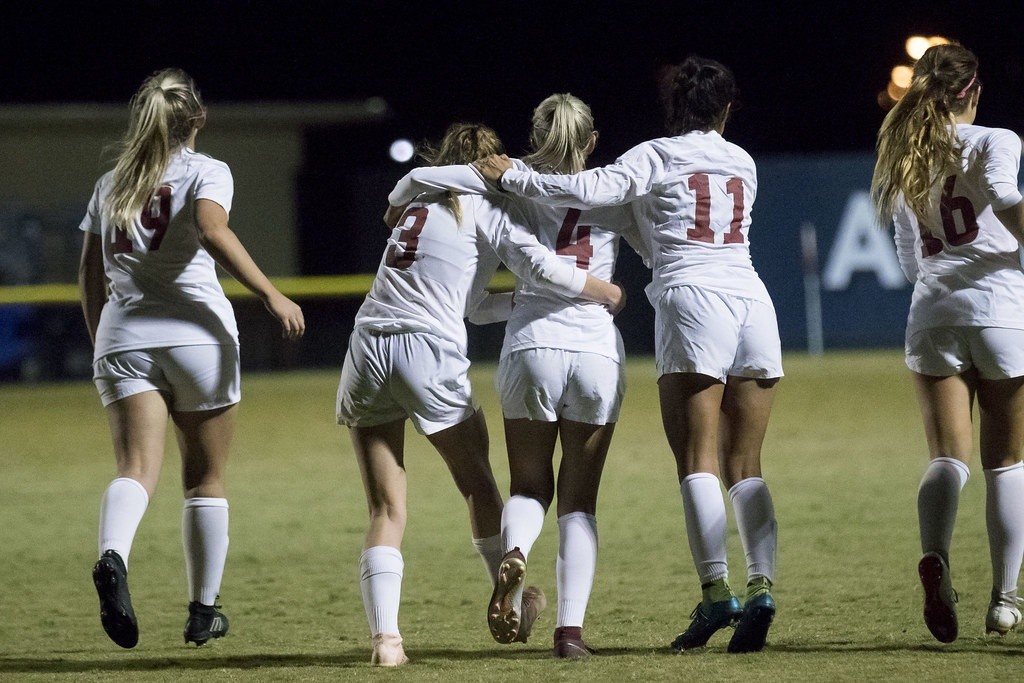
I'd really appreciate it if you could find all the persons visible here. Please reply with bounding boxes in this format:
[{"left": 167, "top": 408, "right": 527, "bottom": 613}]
[
  {"left": 330, "top": 59, "right": 787, "bottom": 666},
  {"left": 873, "top": 41, "right": 1023, "bottom": 642},
  {"left": 81, "top": 68, "right": 306, "bottom": 649}
]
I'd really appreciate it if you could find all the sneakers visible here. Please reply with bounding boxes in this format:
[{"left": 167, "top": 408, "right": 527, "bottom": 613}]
[
  {"left": 183, "top": 595, "right": 229, "bottom": 647},
  {"left": 918, "top": 552, "right": 959, "bottom": 644},
  {"left": 553, "top": 640, "right": 597, "bottom": 658},
  {"left": 727, "top": 592, "right": 776, "bottom": 653},
  {"left": 985, "top": 597, "right": 1024, "bottom": 636},
  {"left": 671, "top": 597, "right": 744, "bottom": 650},
  {"left": 488, "top": 546, "right": 527, "bottom": 644},
  {"left": 513, "top": 585, "right": 547, "bottom": 645},
  {"left": 370, "top": 632, "right": 410, "bottom": 667},
  {"left": 92, "top": 549, "right": 140, "bottom": 649}
]
